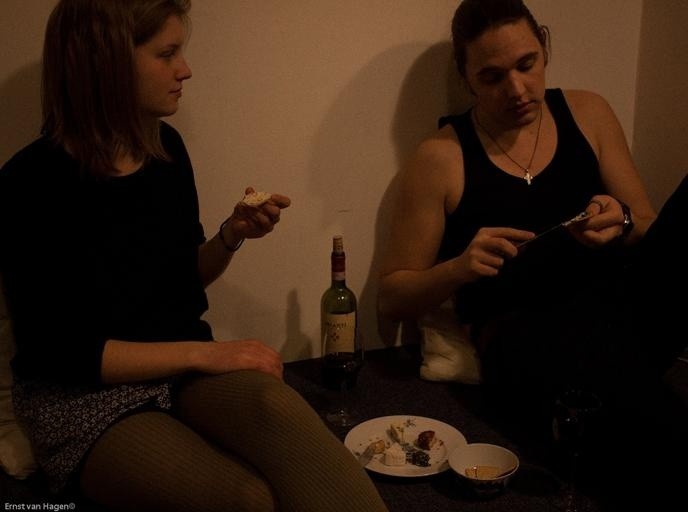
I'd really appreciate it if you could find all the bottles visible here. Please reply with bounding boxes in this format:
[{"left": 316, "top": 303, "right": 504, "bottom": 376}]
[{"left": 319, "top": 237, "right": 357, "bottom": 388}]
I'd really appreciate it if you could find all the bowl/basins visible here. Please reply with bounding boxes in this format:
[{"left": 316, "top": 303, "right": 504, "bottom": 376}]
[{"left": 448, "top": 441, "right": 519, "bottom": 493}]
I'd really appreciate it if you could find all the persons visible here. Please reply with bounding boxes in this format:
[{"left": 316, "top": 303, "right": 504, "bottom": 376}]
[
  {"left": 2, "top": 0, "right": 397, "bottom": 511},
  {"left": 375, "top": 2, "right": 688, "bottom": 512}
]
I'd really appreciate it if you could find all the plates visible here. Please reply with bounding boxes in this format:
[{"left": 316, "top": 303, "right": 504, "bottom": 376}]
[{"left": 343, "top": 413, "right": 468, "bottom": 477}]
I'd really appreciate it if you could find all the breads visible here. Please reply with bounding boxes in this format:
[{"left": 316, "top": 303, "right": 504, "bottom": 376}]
[
  {"left": 418, "top": 431, "right": 436, "bottom": 450},
  {"left": 239, "top": 191, "right": 272, "bottom": 206}
]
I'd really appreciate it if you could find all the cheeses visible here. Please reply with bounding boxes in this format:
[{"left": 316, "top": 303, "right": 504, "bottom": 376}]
[
  {"left": 384, "top": 442, "right": 406, "bottom": 466},
  {"left": 391, "top": 423, "right": 409, "bottom": 442}
]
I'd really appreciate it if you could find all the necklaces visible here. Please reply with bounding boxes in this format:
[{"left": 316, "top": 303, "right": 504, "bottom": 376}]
[{"left": 471, "top": 105, "right": 545, "bottom": 186}]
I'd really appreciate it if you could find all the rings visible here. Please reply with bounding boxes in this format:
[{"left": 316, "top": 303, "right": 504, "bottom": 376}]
[{"left": 588, "top": 199, "right": 605, "bottom": 214}]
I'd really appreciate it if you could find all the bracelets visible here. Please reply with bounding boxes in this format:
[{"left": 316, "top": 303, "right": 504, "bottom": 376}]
[
  {"left": 611, "top": 199, "right": 636, "bottom": 250},
  {"left": 218, "top": 216, "right": 248, "bottom": 252}
]
